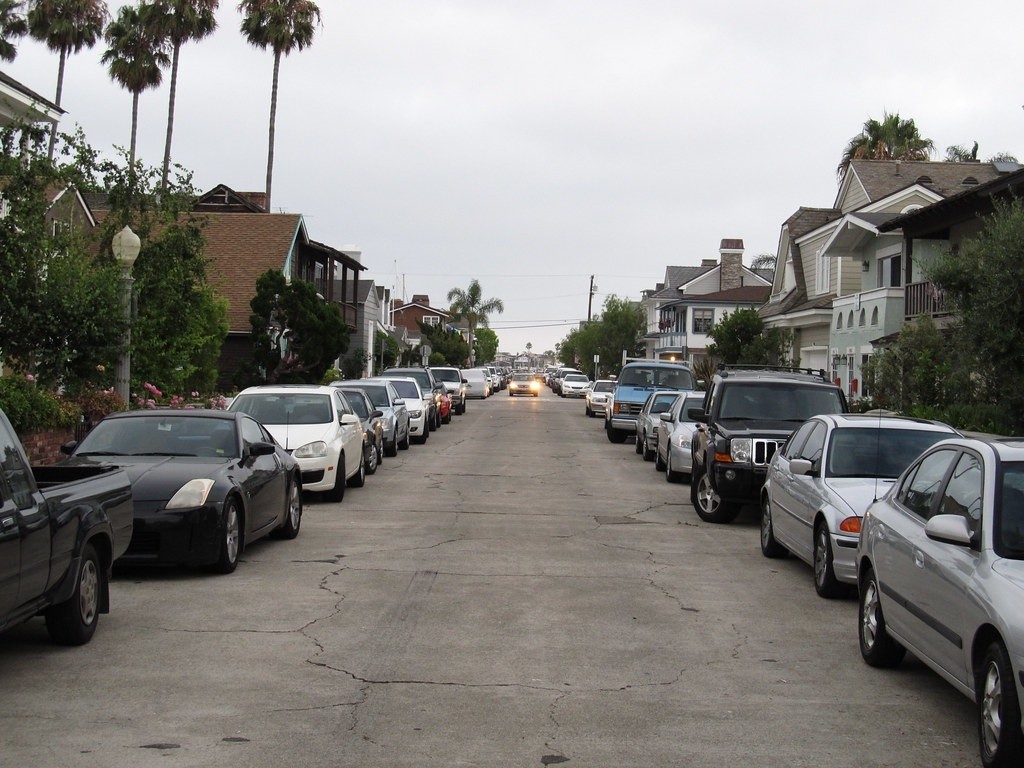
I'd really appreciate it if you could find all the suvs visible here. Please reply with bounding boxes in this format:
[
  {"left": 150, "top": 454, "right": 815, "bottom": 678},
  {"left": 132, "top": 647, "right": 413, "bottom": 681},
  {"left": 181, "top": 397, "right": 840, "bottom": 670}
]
[{"left": 687, "top": 363, "right": 852, "bottom": 525}]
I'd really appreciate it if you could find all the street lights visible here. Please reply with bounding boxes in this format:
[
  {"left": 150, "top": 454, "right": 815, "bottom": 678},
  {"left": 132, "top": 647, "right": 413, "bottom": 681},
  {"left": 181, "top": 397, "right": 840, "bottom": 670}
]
[{"left": 111, "top": 225, "right": 140, "bottom": 411}]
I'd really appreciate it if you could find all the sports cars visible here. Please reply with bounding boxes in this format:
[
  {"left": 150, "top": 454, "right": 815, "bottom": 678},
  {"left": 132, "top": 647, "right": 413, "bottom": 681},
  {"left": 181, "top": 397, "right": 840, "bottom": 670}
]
[{"left": 53, "top": 406, "right": 302, "bottom": 576}]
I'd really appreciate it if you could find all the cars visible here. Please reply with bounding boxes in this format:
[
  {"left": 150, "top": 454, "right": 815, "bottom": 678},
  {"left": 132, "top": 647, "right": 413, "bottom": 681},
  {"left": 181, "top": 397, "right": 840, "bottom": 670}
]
[
  {"left": 508, "top": 371, "right": 538, "bottom": 396},
  {"left": 851, "top": 394, "right": 1024, "bottom": 767},
  {"left": 539, "top": 361, "right": 711, "bottom": 479},
  {"left": 327, "top": 374, "right": 411, "bottom": 455},
  {"left": 360, "top": 375, "right": 434, "bottom": 443},
  {"left": 758, "top": 410, "right": 967, "bottom": 599},
  {"left": 385, "top": 364, "right": 515, "bottom": 431},
  {"left": 324, "top": 387, "right": 385, "bottom": 474},
  {"left": 222, "top": 384, "right": 367, "bottom": 505}
]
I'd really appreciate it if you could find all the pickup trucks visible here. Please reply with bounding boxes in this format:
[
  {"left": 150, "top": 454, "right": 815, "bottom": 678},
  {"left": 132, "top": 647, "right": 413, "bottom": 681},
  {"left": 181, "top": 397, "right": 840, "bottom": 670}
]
[{"left": 0, "top": 405, "right": 135, "bottom": 659}]
